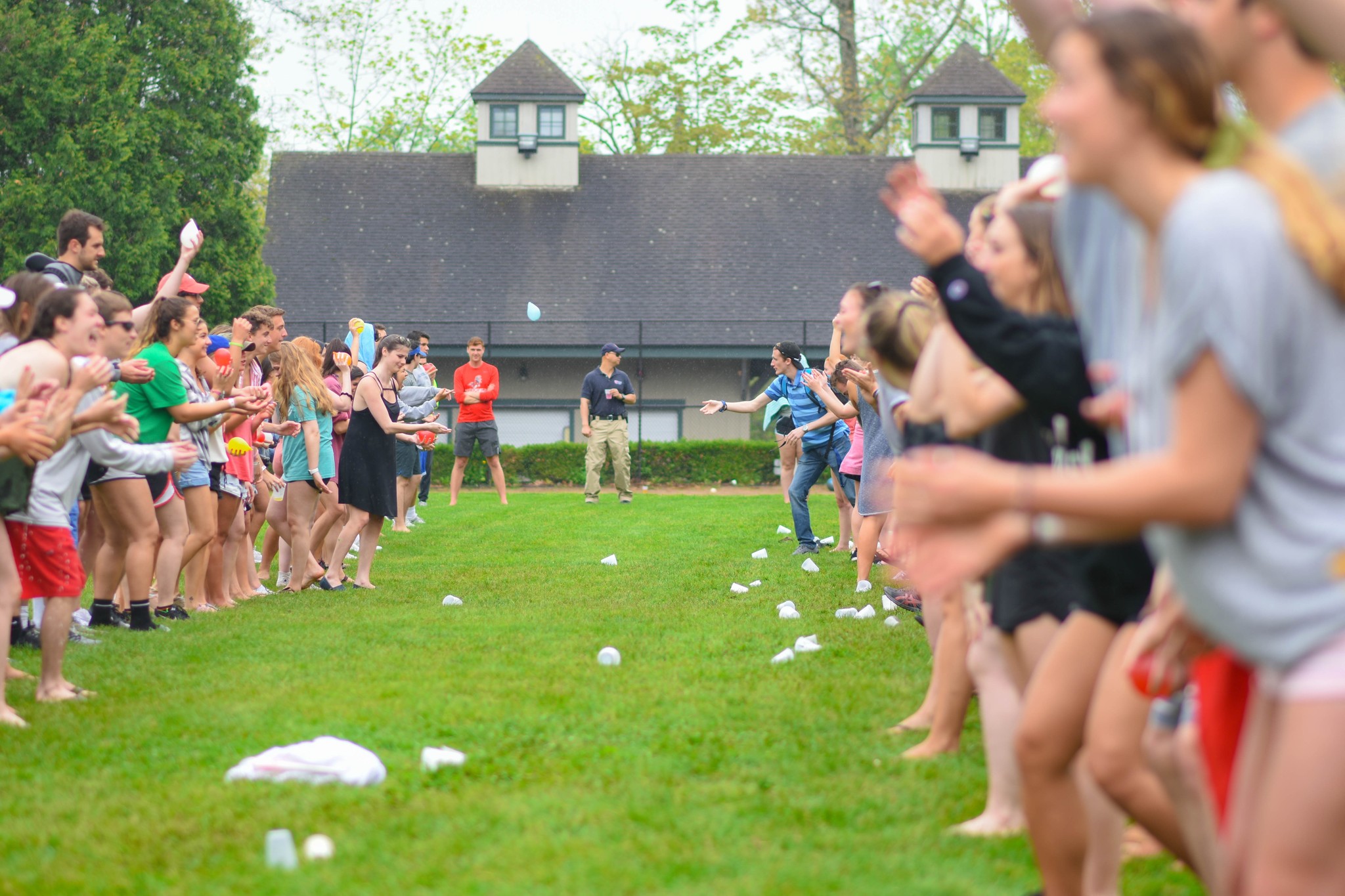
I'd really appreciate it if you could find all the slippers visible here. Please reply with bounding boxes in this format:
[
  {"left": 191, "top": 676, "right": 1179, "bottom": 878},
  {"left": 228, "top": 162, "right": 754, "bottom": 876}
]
[
  {"left": 340, "top": 574, "right": 355, "bottom": 583},
  {"left": 34, "top": 686, "right": 98, "bottom": 704},
  {"left": 204, "top": 602, "right": 222, "bottom": 612},
  {"left": 252, "top": 593, "right": 266, "bottom": 598},
  {"left": 876, "top": 548, "right": 899, "bottom": 568},
  {"left": 885, "top": 725, "right": 930, "bottom": 735},
  {"left": 155, "top": 624, "right": 170, "bottom": 632},
  {"left": 89, "top": 620, "right": 130, "bottom": 629}
]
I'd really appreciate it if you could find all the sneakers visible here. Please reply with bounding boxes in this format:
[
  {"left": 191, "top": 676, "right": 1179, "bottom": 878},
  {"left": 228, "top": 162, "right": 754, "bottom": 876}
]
[
  {"left": 789, "top": 543, "right": 819, "bottom": 555},
  {"left": 884, "top": 585, "right": 922, "bottom": 613}
]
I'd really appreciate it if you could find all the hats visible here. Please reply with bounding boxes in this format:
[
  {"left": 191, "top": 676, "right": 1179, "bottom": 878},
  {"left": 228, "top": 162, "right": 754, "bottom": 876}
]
[
  {"left": 408, "top": 345, "right": 426, "bottom": 356},
  {"left": 206, "top": 335, "right": 230, "bottom": 354},
  {"left": 218, "top": 333, "right": 256, "bottom": 352},
  {"left": 601, "top": 343, "right": 626, "bottom": 356},
  {"left": 157, "top": 271, "right": 209, "bottom": 294},
  {"left": 774, "top": 341, "right": 804, "bottom": 370}
]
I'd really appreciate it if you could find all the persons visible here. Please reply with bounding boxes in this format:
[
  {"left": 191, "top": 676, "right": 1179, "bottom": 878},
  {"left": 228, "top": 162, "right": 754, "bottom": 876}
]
[
  {"left": 701, "top": 0, "right": 1345, "bottom": 896},
  {"left": 0, "top": 208, "right": 507, "bottom": 731},
  {"left": 580, "top": 344, "right": 635, "bottom": 502}
]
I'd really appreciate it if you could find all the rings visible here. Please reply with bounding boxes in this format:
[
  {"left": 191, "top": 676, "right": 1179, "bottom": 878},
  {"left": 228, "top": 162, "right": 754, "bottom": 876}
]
[
  {"left": 272, "top": 482, "right": 275, "bottom": 485},
  {"left": 320, "top": 487, "right": 323, "bottom": 491}
]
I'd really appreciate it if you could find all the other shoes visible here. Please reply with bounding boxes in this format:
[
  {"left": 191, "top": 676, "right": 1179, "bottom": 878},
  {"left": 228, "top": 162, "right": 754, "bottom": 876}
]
[
  {"left": 892, "top": 569, "right": 910, "bottom": 581},
  {"left": 849, "top": 547, "right": 858, "bottom": 561},
  {"left": 23, "top": 620, "right": 40, "bottom": 639},
  {"left": 307, "top": 583, "right": 323, "bottom": 591},
  {"left": 318, "top": 558, "right": 346, "bottom": 570},
  {"left": 68, "top": 630, "right": 102, "bottom": 645},
  {"left": 87, "top": 602, "right": 129, "bottom": 621},
  {"left": 409, "top": 513, "right": 425, "bottom": 523},
  {"left": 391, "top": 519, "right": 415, "bottom": 527},
  {"left": 154, "top": 604, "right": 191, "bottom": 621},
  {"left": 11, "top": 634, "right": 41, "bottom": 650},
  {"left": 276, "top": 570, "right": 292, "bottom": 587},
  {"left": 173, "top": 593, "right": 185, "bottom": 607},
  {"left": 915, "top": 614, "right": 924, "bottom": 626},
  {"left": 319, "top": 576, "right": 346, "bottom": 592},
  {"left": 353, "top": 583, "right": 381, "bottom": 590}
]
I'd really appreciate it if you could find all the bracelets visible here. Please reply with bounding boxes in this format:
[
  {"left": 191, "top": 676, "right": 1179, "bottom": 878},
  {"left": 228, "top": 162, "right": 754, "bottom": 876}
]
[
  {"left": 1025, "top": 510, "right": 1067, "bottom": 545},
  {"left": 341, "top": 392, "right": 353, "bottom": 401},
  {"left": 719, "top": 401, "right": 727, "bottom": 412},
  {"left": 229, "top": 398, "right": 235, "bottom": 408},
  {"left": 253, "top": 449, "right": 259, "bottom": 459},
  {"left": 262, "top": 465, "right": 266, "bottom": 472},
  {"left": 872, "top": 389, "right": 880, "bottom": 401},
  {"left": 247, "top": 415, "right": 250, "bottom": 419},
  {"left": 230, "top": 342, "right": 243, "bottom": 348},
  {"left": 254, "top": 461, "right": 261, "bottom": 465}
]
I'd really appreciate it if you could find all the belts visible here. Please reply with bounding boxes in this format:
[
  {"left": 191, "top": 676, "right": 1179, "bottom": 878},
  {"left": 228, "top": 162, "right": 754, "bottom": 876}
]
[{"left": 590, "top": 415, "right": 623, "bottom": 420}]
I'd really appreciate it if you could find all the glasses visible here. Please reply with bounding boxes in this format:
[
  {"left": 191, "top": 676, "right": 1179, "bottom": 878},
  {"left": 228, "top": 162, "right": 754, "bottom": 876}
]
[
  {"left": 182, "top": 318, "right": 202, "bottom": 326},
  {"left": 782, "top": 352, "right": 801, "bottom": 362},
  {"left": 395, "top": 336, "right": 411, "bottom": 347},
  {"left": 609, "top": 353, "right": 621, "bottom": 357},
  {"left": 184, "top": 293, "right": 199, "bottom": 301},
  {"left": 834, "top": 364, "right": 842, "bottom": 371},
  {"left": 866, "top": 280, "right": 881, "bottom": 291},
  {"left": 106, "top": 321, "right": 135, "bottom": 332}
]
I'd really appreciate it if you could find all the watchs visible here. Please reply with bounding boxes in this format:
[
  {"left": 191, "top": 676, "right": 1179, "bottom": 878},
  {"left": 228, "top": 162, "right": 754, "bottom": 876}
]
[
  {"left": 210, "top": 389, "right": 223, "bottom": 400},
  {"left": 619, "top": 393, "right": 625, "bottom": 401},
  {"left": 802, "top": 425, "right": 808, "bottom": 433},
  {"left": 307, "top": 468, "right": 319, "bottom": 475}
]
